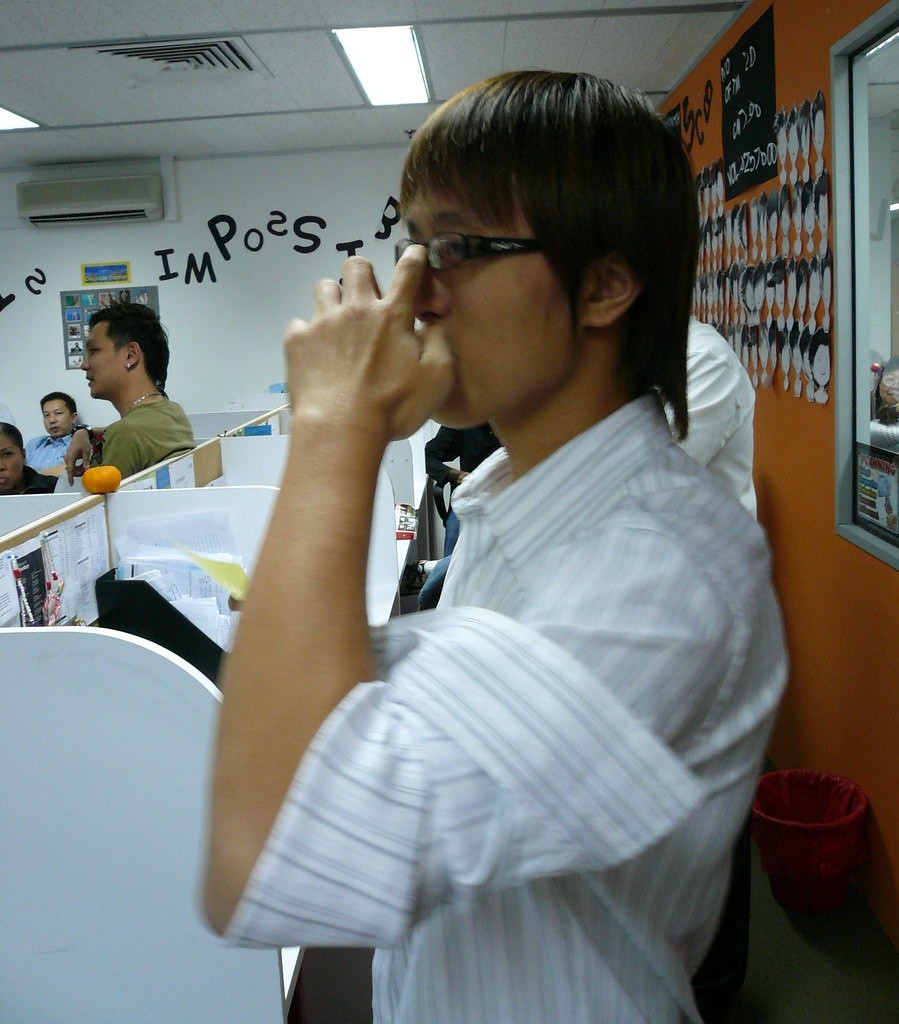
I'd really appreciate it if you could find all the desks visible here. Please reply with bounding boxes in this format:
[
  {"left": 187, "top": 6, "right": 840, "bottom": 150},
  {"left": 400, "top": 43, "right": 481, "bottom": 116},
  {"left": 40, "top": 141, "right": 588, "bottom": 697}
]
[{"left": 0, "top": 406, "right": 435, "bottom": 1023}]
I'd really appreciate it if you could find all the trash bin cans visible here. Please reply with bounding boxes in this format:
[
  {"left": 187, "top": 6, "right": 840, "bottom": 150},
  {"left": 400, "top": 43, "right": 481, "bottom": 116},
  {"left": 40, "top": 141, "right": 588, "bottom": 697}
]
[{"left": 750, "top": 770, "right": 868, "bottom": 921}]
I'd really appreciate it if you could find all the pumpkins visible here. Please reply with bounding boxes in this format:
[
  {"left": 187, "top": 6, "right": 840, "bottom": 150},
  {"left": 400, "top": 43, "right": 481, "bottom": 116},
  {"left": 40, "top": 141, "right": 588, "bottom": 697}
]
[{"left": 81, "top": 465, "right": 121, "bottom": 494}]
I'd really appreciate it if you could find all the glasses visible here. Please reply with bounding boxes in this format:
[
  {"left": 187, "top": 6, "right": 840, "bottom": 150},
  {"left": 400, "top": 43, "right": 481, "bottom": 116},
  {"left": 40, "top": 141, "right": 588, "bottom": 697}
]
[{"left": 395, "top": 234, "right": 549, "bottom": 270}]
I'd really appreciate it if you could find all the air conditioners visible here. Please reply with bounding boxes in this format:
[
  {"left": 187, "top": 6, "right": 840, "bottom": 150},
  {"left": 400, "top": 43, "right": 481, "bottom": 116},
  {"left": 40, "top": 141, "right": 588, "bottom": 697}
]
[{"left": 16, "top": 173, "right": 164, "bottom": 229}]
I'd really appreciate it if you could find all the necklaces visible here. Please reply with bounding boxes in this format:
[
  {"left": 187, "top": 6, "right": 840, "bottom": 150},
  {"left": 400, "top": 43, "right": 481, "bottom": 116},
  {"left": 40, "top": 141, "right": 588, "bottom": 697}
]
[{"left": 123, "top": 392, "right": 163, "bottom": 413}]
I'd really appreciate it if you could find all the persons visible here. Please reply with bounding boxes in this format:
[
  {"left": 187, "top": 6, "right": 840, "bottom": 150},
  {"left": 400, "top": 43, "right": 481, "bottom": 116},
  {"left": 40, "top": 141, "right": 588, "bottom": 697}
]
[
  {"left": 65, "top": 301, "right": 195, "bottom": 487},
  {"left": 0, "top": 422, "right": 58, "bottom": 494},
  {"left": 202, "top": 71, "right": 790, "bottom": 1023},
  {"left": 24, "top": 392, "right": 78, "bottom": 475}
]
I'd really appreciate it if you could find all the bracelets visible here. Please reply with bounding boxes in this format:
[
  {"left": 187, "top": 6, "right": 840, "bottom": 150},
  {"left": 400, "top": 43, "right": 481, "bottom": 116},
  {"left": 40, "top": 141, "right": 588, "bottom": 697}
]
[{"left": 70, "top": 424, "right": 92, "bottom": 446}]
